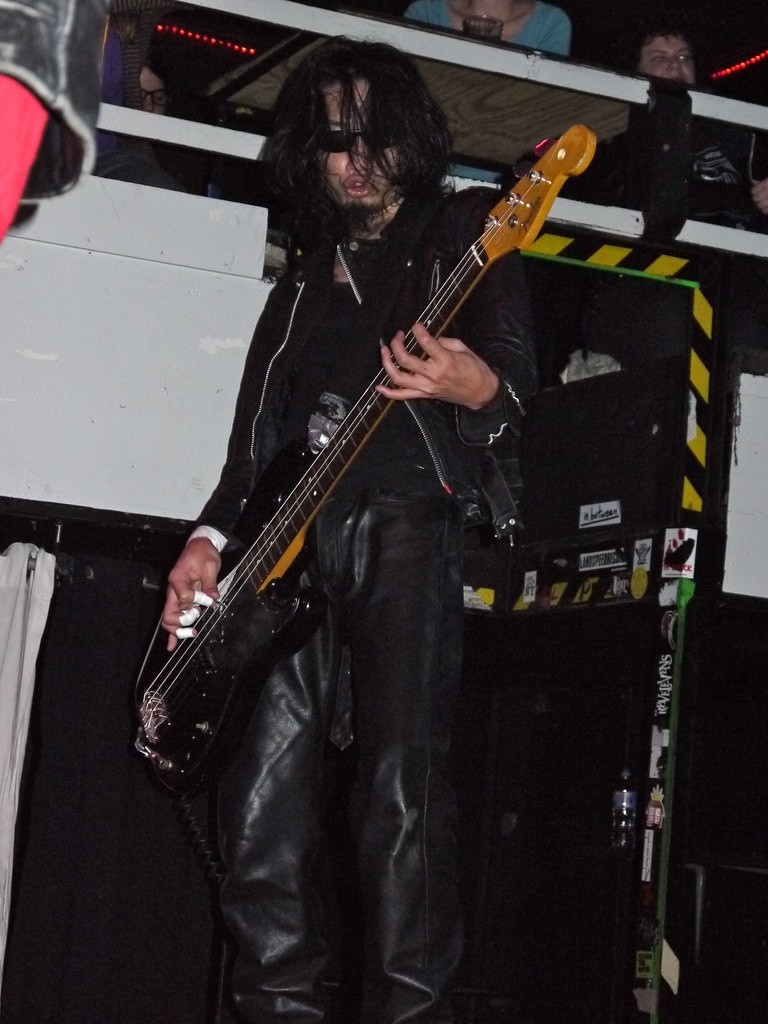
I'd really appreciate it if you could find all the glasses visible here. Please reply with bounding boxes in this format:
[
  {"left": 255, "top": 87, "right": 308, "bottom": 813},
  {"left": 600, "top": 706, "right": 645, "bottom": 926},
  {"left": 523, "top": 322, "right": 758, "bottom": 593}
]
[{"left": 320, "top": 129, "right": 395, "bottom": 152}]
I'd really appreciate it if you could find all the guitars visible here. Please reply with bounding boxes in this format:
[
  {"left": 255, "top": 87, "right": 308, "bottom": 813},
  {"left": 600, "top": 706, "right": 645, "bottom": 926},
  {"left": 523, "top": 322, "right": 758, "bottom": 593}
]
[{"left": 126, "top": 120, "right": 601, "bottom": 781}]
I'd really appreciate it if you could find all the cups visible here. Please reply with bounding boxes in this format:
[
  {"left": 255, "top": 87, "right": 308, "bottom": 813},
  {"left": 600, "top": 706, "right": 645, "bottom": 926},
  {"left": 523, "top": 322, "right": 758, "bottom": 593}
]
[{"left": 464, "top": 13, "right": 504, "bottom": 41}]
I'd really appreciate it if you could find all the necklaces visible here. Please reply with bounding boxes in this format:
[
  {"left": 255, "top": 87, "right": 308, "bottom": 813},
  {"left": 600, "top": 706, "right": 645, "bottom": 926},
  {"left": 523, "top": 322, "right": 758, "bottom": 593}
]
[{"left": 372, "top": 203, "right": 396, "bottom": 215}]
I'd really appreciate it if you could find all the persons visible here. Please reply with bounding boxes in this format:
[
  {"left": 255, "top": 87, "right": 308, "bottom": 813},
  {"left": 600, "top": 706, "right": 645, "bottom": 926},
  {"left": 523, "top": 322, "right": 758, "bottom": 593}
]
[
  {"left": 605, "top": 9, "right": 768, "bottom": 234},
  {"left": 158, "top": 38, "right": 534, "bottom": 1024},
  {"left": 140, "top": 61, "right": 178, "bottom": 115},
  {"left": 404, "top": 0, "right": 571, "bottom": 59}
]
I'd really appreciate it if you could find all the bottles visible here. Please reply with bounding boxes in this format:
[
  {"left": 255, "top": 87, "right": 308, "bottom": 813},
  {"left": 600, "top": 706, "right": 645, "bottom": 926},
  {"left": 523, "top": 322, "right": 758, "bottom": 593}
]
[{"left": 611, "top": 766, "right": 636, "bottom": 851}]
